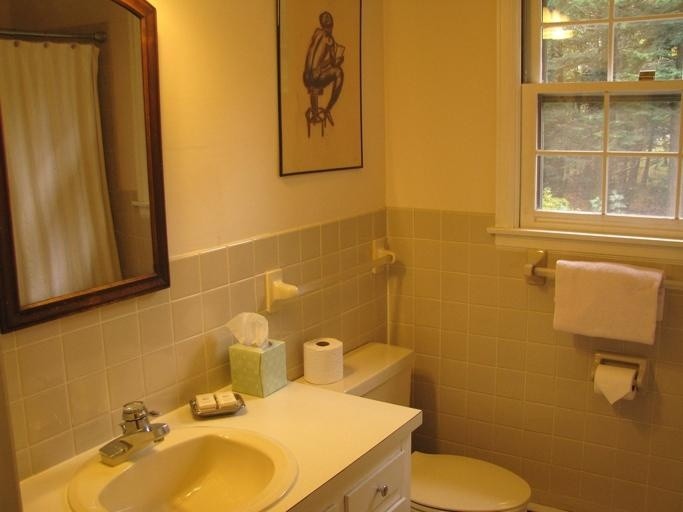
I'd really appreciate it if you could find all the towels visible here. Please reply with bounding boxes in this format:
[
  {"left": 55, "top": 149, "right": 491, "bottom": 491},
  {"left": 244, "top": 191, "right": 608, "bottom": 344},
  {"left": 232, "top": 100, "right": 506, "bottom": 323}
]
[{"left": 550, "top": 258, "right": 668, "bottom": 348}]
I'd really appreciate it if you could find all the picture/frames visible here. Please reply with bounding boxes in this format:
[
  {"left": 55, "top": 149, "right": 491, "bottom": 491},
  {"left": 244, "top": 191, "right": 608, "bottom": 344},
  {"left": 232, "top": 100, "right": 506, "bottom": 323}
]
[{"left": 274, "top": 0, "right": 364, "bottom": 178}]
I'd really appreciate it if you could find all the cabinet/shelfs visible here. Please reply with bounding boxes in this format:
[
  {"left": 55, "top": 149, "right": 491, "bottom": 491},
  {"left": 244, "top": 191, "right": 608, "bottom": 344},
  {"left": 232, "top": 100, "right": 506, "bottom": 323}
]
[{"left": 343, "top": 448, "right": 408, "bottom": 512}]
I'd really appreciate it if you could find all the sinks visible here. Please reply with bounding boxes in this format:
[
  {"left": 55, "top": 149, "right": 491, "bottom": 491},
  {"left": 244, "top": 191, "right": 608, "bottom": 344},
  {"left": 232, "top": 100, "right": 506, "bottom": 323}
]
[{"left": 63, "top": 424, "right": 299, "bottom": 512}]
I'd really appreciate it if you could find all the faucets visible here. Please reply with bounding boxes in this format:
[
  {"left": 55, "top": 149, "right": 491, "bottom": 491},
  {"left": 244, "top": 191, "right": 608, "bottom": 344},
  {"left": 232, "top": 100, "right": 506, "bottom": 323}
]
[{"left": 96, "top": 397, "right": 171, "bottom": 469}]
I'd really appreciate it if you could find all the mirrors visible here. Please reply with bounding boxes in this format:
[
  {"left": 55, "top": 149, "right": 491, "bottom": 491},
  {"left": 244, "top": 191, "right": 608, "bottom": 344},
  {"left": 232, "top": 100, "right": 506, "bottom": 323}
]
[{"left": 0, "top": 0, "right": 172, "bottom": 337}]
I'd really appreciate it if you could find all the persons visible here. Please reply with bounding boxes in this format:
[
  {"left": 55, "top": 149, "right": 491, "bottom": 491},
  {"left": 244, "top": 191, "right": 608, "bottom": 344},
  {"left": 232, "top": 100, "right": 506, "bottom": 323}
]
[{"left": 303, "top": 12, "right": 343, "bottom": 126}]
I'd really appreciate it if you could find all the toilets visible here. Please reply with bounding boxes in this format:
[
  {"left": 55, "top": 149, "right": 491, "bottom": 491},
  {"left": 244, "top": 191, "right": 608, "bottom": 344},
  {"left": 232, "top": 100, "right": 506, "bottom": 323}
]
[{"left": 293, "top": 342, "right": 534, "bottom": 510}]
